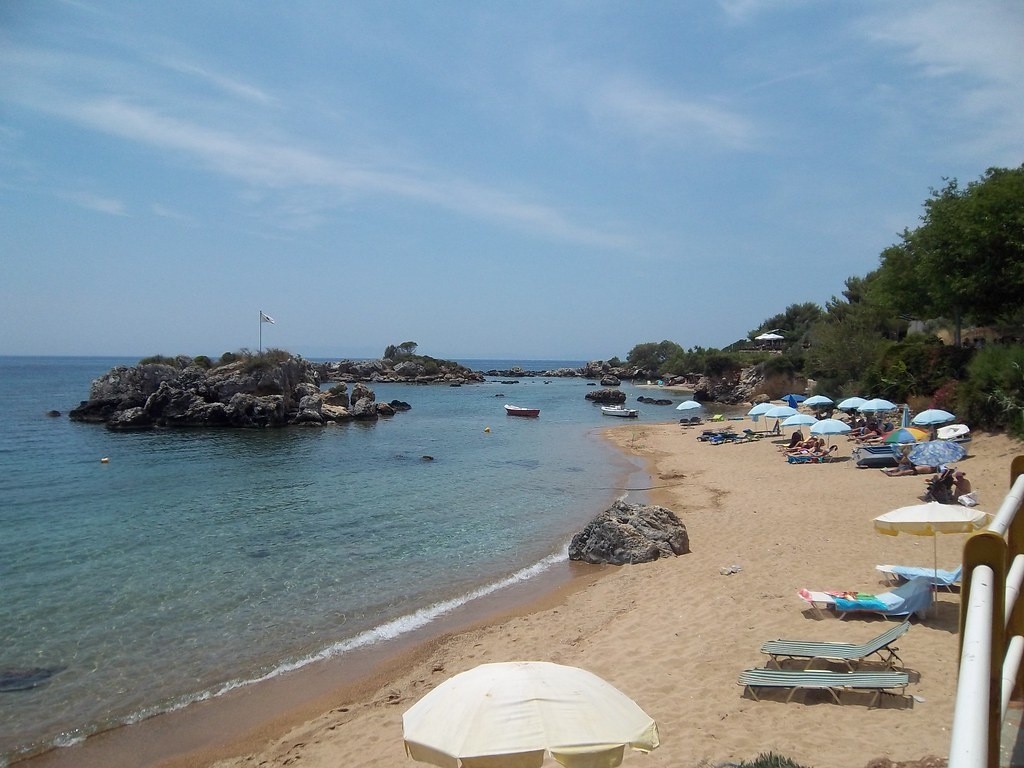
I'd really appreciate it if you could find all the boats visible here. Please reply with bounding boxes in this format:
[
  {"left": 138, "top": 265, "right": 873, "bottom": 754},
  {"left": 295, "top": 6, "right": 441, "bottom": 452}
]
[
  {"left": 504, "top": 404, "right": 540, "bottom": 417},
  {"left": 851, "top": 435, "right": 972, "bottom": 469},
  {"left": 601, "top": 404, "right": 639, "bottom": 417}
]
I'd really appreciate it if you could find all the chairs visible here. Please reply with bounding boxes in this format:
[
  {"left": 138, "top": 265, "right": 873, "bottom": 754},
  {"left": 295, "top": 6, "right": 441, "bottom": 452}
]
[
  {"left": 797, "top": 576, "right": 933, "bottom": 623},
  {"left": 760, "top": 621, "right": 911, "bottom": 672},
  {"left": 736, "top": 668, "right": 909, "bottom": 711},
  {"left": 876, "top": 564, "right": 962, "bottom": 594},
  {"left": 678, "top": 415, "right": 883, "bottom": 464}
]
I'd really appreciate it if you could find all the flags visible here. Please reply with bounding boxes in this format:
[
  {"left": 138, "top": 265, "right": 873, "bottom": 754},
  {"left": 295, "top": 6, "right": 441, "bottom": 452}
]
[{"left": 261, "top": 312, "right": 277, "bottom": 326}]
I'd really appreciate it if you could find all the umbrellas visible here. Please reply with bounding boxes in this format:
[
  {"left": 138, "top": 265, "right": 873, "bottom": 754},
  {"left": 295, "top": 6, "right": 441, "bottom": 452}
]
[
  {"left": 764, "top": 406, "right": 799, "bottom": 439},
  {"left": 809, "top": 419, "right": 851, "bottom": 449},
  {"left": 802, "top": 395, "right": 833, "bottom": 419},
  {"left": 873, "top": 500, "right": 992, "bottom": 621},
  {"left": 911, "top": 409, "right": 955, "bottom": 441},
  {"left": 881, "top": 427, "right": 929, "bottom": 444},
  {"left": 747, "top": 403, "right": 776, "bottom": 431},
  {"left": 857, "top": 398, "right": 897, "bottom": 417},
  {"left": 402, "top": 661, "right": 661, "bottom": 768},
  {"left": 907, "top": 440, "right": 965, "bottom": 466},
  {"left": 837, "top": 397, "right": 867, "bottom": 418},
  {"left": 781, "top": 393, "right": 807, "bottom": 408},
  {"left": 779, "top": 415, "right": 819, "bottom": 430},
  {"left": 676, "top": 400, "right": 701, "bottom": 410}
]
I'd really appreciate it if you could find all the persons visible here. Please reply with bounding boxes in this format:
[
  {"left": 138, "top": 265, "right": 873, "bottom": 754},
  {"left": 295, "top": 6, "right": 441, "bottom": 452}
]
[
  {"left": 788, "top": 430, "right": 804, "bottom": 448},
  {"left": 788, "top": 430, "right": 833, "bottom": 457},
  {"left": 845, "top": 413, "right": 894, "bottom": 442},
  {"left": 952, "top": 472, "right": 971, "bottom": 502},
  {"left": 881, "top": 448, "right": 936, "bottom": 477}
]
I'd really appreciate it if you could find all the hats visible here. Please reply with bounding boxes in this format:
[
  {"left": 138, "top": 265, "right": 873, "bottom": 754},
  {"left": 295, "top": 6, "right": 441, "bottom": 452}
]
[{"left": 953, "top": 472, "right": 963, "bottom": 476}]
